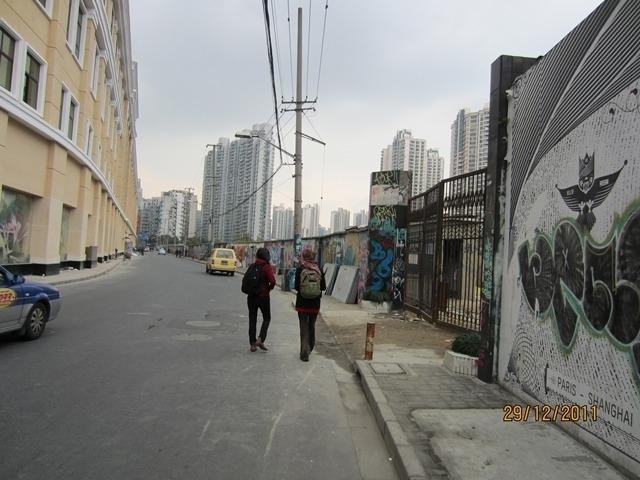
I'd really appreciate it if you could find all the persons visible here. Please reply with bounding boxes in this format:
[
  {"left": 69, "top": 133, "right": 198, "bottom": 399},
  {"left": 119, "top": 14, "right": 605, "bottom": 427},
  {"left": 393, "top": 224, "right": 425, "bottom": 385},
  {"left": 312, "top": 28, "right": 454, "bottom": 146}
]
[
  {"left": 175, "top": 247, "right": 182, "bottom": 259},
  {"left": 241, "top": 246, "right": 276, "bottom": 353},
  {"left": 293, "top": 248, "right": 326, "bottom": 361}
]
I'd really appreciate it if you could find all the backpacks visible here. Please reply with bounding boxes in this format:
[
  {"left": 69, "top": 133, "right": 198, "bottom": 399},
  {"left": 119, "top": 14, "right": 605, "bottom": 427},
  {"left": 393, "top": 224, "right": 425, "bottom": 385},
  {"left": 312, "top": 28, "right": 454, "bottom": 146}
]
[
  {"left": 242, "top": 263, "right": 270, "bottom": 296},
  {"left": 300, "top": 264, "right": 322, "bottom": 299}
]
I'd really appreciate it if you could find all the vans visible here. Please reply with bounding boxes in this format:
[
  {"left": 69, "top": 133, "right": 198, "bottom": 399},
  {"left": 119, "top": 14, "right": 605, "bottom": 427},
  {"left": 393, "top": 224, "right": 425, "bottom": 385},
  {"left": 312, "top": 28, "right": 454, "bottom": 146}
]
[{"left": 206, "top": 247, "right": 237, "bottom": 275}]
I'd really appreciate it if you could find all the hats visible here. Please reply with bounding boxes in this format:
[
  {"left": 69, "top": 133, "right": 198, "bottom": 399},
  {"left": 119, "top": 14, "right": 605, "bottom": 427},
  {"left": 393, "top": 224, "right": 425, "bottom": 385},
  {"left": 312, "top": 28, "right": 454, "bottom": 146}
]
[
  {"left": 299, "top": 249, "right": 315, "bottom": 260},
  {"left": 257, "top": 248, "right": 270, "bottom": 259}
]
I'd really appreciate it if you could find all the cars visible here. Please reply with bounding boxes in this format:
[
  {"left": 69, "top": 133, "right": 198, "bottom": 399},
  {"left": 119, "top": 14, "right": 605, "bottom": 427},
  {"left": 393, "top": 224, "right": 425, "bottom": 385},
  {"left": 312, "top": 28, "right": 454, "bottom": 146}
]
[
  {"left": 1, "top": 264, "right": 61, "bottom": 339},
  {"left": 158, "top": 247, "right": 167, "bottom": 255},
  {"left": 144, "top": 245, "right": 150, "bottom": 252}
]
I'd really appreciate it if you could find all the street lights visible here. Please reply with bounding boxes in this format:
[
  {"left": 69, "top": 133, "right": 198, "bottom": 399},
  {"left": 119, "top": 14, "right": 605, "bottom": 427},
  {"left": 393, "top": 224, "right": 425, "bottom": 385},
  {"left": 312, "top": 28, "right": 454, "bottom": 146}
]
[{"left": 234, "top": 133, "right": 303, "bottom": 266}]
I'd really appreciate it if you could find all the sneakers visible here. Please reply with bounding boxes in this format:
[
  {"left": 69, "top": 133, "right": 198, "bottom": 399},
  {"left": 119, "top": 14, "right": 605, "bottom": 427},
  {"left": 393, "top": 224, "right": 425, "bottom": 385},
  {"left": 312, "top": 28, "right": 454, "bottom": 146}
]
[
  {"left": 303, "top": 355, "right": 309, "bottom": 360},
  {"left": 250, "top": 345, "right": 257, "bottom": 352},
  {"left": 255, "top": 340, "right": 268, "bottom": 350}
]
